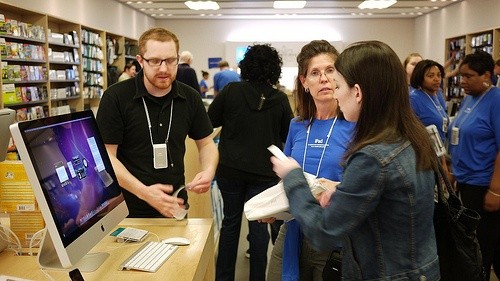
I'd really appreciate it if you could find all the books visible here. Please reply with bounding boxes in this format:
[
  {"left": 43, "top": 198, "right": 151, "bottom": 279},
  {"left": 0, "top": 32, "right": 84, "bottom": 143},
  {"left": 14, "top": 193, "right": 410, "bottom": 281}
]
[
  {"left": 82, "top": 30, "right": 104, "bottom": 100},
  {"left": 0, "top": 13, "right": 80, "bottom": 152}
]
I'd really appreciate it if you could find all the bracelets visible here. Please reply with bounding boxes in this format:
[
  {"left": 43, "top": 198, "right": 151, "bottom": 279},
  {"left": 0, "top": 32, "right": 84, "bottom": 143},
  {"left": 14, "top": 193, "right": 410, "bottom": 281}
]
[
  {"left": 488, "top": 189, "right": 500, "bottom": 197},
  {"left": 449, "top": 57, "right": 453, "bottom": 63}
]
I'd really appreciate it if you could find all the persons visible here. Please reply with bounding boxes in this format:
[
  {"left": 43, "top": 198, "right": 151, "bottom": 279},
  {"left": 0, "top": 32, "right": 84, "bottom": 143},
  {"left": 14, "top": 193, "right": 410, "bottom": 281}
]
[
  {"left": 402, "top": 49, "right": 462, "bottom": 176},
  {"left": 447, "top": 50, "right": 500, "bottom": 281},
  {"left": 214, "top": 59, "right": 242, "bottom": 99},
  {"left": 176, "top": 51, "right": 202, "bottom": 96},
  {"left": 199, "top": 71, "right": 214, "bottom": 98},
  {"left": 267, "top": 38, "right": 358, "bottom": 281},
  {"left": 96, "top": 28, "right": 219, "bottom": 218},
  {"left": 208, "top": 42, "right": 295, "bottom": 280},
  {"left": 258, "top": 40, "right": 443, "bottom": 281},
  {"left": 117, "top": 64, "right": 137, "bottom": 83}
]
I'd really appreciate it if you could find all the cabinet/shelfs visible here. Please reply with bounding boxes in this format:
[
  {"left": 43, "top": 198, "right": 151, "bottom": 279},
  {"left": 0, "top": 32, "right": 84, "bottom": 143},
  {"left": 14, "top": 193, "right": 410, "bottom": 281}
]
[
  {"left": 444, "top": 27, "right": 500, "bottom": 113},
  {"left": 0, "top": 0, "right": 139, "bottom": 117}
]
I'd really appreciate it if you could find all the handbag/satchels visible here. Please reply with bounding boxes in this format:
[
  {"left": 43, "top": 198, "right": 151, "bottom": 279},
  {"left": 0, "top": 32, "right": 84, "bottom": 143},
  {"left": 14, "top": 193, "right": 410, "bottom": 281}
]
[
  {"left": 320, "top": 251, "right": 342, "bottom": 281},
  {"left": 429, "top": 148, "right": 483, "bottom": 281}
]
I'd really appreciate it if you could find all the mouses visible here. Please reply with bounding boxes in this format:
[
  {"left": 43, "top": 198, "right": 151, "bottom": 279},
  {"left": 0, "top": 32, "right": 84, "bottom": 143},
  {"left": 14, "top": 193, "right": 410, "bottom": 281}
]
[{"left": 162, "top": 236, "right": 190, "bottom": 246}]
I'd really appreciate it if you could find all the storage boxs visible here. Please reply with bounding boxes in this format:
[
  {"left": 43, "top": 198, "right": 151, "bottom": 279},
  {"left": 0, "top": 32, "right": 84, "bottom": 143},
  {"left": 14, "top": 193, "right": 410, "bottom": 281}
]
[{"left": 0, "top": 160, "right": 46, "bottom": 248}]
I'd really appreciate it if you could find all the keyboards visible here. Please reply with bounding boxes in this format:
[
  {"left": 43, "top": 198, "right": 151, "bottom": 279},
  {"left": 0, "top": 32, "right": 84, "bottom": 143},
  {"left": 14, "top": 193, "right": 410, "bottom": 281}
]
[{"left": 119, "top": 240, "right": 179, "bottom": 272}]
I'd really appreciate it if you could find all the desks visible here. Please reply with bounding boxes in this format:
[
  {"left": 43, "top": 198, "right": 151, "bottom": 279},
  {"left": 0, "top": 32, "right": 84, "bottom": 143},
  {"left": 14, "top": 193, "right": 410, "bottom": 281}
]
[{"left": 0, "top": 218, "right": 216, "bottom": 281}]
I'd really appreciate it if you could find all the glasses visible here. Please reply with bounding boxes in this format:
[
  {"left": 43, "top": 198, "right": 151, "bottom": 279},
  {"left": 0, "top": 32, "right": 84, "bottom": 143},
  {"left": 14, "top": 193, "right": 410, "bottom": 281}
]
[{"left": 140, "top": 54, "right": 179, "bottom": 67}]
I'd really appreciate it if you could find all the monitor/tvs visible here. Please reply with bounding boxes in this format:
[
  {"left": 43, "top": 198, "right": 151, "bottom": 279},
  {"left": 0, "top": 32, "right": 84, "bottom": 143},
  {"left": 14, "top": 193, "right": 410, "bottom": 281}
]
[{"left": 8, "top": 110, "right": 130, "bottom": 272}]
